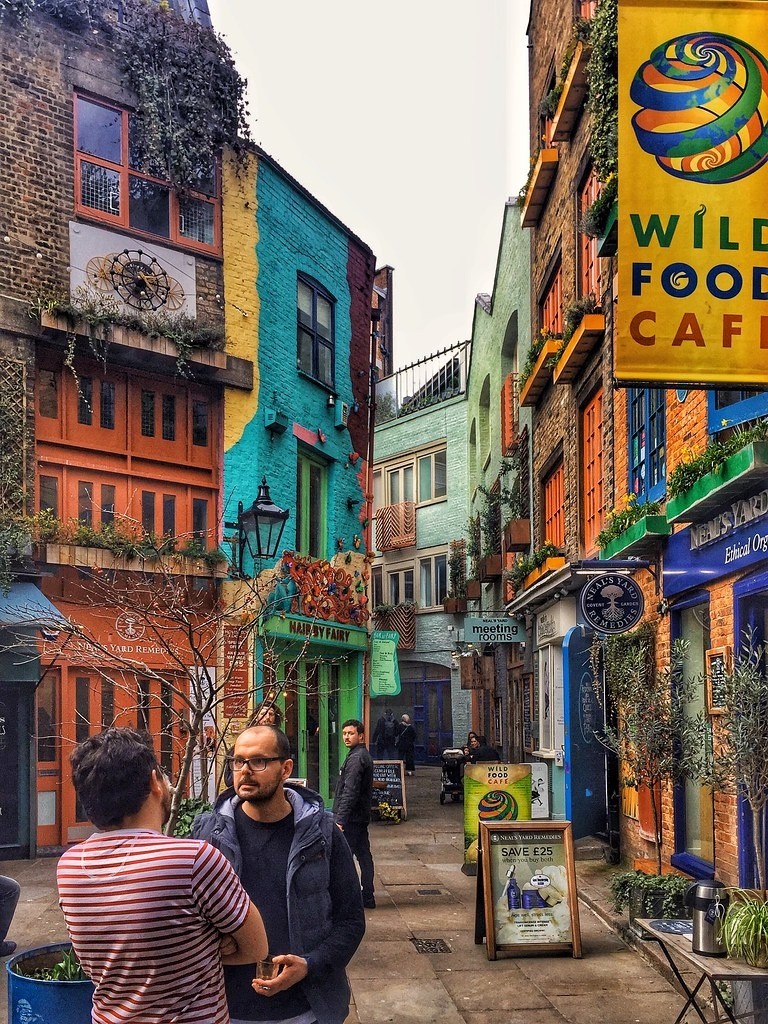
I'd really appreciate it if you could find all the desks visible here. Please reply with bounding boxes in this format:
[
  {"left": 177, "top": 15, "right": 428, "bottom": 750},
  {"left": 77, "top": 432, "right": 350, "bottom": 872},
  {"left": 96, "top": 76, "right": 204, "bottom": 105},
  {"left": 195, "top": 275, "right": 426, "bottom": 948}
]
[{"left": 634, "top": 918, "right": 768, "bottom": 1024}]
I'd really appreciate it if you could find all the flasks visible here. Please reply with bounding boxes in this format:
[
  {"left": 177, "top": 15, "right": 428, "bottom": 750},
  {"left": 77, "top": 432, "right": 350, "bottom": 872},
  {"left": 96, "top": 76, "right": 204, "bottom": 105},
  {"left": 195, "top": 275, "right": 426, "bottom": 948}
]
[{"left": 683, "top": 879, "right": 729, "bottom": 957}]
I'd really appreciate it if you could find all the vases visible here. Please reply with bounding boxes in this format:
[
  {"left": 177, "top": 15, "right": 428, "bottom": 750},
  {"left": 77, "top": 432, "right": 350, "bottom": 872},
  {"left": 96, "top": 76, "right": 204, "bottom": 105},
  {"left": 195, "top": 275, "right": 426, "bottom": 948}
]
[
  {"left": 601, "top": 515, "right": 672, "bottom": 561},
  {"left": 520, "top": 148, "right": 558, "bottom": 228},
  {"left": 596, "top": 204, "right": 618, "bottom": 257},
  {"left": 553, "top": 314, "right": 605, "bottom": 385},
  {"left": 522, "top": 557, "right": 565, "bottom": 591},
  {"left": 550, "top": 42, "right": 593, "bottom": 143},
  {"left": 666, "top": 441, "right": 768, "bottom": 524},
  {"left": 519, "top": 339, "right": 564, "bottom": 407}
]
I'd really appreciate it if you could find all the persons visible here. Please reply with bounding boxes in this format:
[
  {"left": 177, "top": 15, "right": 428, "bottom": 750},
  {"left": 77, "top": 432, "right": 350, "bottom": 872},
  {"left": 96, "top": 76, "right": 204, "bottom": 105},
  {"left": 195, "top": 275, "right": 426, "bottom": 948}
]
[
  {"left": 461, "top": 731, "right": 500, "bottom": 764},
  {"left": 372, "top": 709, "right": 399, "bottom": 759},
  {"left": 224, "top": 701, "right": 283, "bottom": 786},
  {"left": 57, "top": 727, "right": 269, "bottom": 1024},
  {"left": 396, "top": 714, "right": 417, "bottom": 776},
  {"left": 0, "top": 875, "right": 21, "bottom": 955},
  {"left": 331, "top": 719, "right": 376, "bottom": 909},
  {"left": 190, "top": 724, "right": 366, "bottom": 1024}
]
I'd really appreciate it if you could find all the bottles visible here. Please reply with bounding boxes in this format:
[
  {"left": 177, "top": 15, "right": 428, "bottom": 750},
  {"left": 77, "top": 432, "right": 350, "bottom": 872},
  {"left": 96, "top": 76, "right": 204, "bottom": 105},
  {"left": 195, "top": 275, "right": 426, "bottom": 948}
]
[{"left": 507, "top": 879, "right": 521, "bottom": 910}]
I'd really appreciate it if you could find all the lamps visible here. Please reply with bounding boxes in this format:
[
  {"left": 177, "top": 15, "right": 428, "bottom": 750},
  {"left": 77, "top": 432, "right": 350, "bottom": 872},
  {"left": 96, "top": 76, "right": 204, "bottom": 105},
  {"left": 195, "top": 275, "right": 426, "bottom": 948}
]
[
  {"left": 230, "top": 476, "right": 289, "bottom": 580},
  {"left": 451, "top": 641, "right": 472, "bottom": 658}
]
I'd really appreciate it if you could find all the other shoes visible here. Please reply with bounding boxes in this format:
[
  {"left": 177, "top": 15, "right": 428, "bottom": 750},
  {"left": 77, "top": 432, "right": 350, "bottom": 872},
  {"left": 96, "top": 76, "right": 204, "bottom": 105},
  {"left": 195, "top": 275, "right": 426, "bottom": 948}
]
[
  {"left": 365, "top": 900, "right": 376, "bottom": 908},
  {"left": 0, "top": 941, "right": 17, "bottom": 955},
  {"left": 407, "top": 771, "right": 412, "bottom": 775}
]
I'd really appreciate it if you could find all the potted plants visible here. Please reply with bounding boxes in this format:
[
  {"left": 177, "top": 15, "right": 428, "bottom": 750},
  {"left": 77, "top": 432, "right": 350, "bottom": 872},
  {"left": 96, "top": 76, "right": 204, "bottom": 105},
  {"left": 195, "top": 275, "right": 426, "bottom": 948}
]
[
  {"left": 6, "top": 942, "right": 96, "bottom": 1024},
  {"left": 592, "top": 618, "right": 768, "bottom": 1024},
  {"left": 443, "top": 454, "right": 530, "bottom": 615},
  {"left": 26, "top": 285, "right": 233, "bottom": 413},
  {"left": 33, "top": 508, "right": 229, "bottom": 579}
]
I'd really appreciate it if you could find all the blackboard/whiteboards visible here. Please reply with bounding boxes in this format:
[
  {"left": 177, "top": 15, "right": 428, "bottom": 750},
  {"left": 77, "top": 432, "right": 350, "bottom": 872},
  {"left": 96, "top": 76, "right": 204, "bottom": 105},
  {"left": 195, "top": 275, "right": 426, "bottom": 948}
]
[{"left": 371, "top": 760, "right": 406, "bottom": 809}]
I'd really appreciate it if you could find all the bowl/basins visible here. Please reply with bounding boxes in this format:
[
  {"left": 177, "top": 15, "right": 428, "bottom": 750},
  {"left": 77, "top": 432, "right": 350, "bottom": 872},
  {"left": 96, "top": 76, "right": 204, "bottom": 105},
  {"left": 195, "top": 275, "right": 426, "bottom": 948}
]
[{"left": 523, "top": 890, "right": 548, "bottom": 910}]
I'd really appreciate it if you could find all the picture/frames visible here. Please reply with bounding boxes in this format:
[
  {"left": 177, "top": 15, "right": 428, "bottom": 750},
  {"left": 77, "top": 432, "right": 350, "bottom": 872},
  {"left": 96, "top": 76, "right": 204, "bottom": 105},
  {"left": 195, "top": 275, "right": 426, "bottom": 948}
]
[{"left": 705, "top": 646, "right": 731, "bottom": 714}]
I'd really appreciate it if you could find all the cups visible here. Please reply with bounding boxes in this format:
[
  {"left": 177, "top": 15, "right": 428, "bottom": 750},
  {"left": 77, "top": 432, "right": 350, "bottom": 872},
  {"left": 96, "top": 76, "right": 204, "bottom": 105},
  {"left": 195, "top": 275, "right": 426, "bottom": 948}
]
[{"left": 256, "top": 954, "right": 280, "bottom": 990}]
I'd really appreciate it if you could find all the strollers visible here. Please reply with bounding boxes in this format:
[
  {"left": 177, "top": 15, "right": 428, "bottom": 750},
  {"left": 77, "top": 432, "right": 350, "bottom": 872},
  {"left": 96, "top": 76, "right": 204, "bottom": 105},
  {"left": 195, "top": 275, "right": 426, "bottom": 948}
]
[{"left": 437, "top": 747, "right": 472, "bottom": 804}]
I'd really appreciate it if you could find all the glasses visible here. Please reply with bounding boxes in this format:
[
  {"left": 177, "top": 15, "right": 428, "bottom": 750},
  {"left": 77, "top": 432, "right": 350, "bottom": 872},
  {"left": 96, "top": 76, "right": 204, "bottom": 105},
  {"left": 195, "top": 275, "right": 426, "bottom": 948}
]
[{"left": 228, "top": 757, "right": 284, "bottom": 772}]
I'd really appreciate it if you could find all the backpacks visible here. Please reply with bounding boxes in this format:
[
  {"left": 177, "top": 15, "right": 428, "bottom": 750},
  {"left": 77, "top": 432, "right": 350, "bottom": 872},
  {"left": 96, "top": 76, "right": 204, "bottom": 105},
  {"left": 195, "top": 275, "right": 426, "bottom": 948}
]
[{"left": 381, "top": 716, "right": 396, "bottom": 743}]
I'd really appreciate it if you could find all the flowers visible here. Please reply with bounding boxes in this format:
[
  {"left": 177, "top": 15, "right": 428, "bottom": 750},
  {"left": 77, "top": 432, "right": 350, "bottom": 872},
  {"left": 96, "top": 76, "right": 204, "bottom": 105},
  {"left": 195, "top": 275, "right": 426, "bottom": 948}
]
[
  {"left": 665, "top": 412, "right": 768, "bottom": 501},
  {"left": 578, "top": 171, "right": 618, "bottom": 239},
  {"left": 517, "top": 296, "right": 594, "bottom": 394},
  {"left": 594, "top": 493, "right": 661, "bottom": 549},
  {"left": 509, "top": 540, "right": 559, "bottom": 581},
  {"left": 515, "top": 134, "right": 546, "bottom": 214},
  {"left": 547, "top": 36, "right": 578, "bottom": 115}
]
